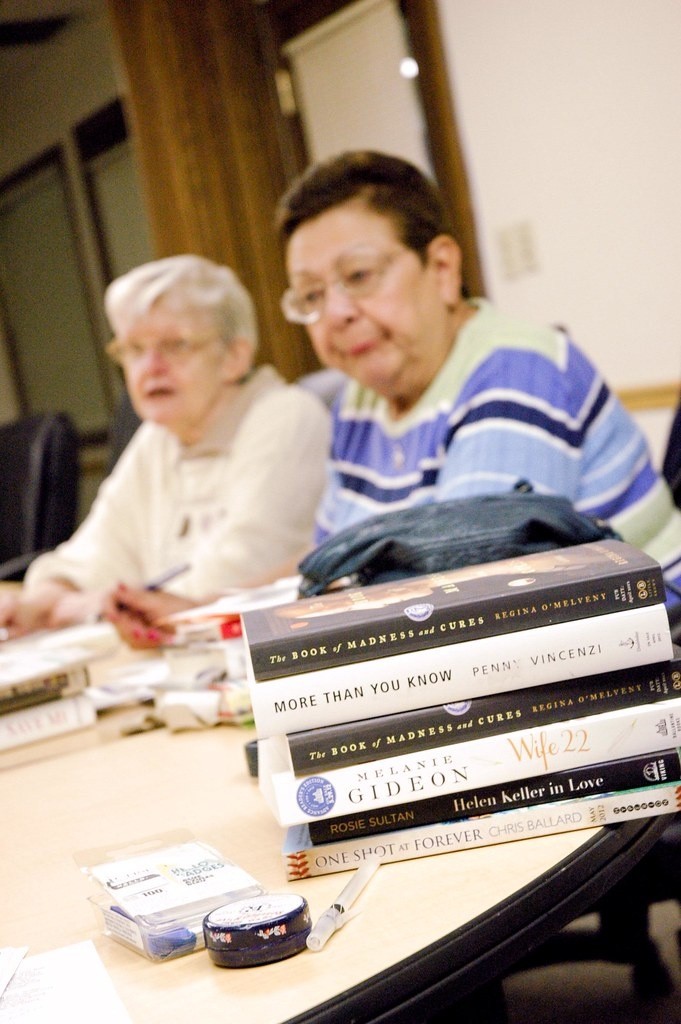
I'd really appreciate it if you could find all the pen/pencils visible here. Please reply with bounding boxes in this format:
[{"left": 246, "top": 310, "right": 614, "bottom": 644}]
[
  {"left": 92, "top": 564, "right": 189, "bottom": 623},
  {"left": 307, "top": 854, "right": 379, "bottom": 951}
]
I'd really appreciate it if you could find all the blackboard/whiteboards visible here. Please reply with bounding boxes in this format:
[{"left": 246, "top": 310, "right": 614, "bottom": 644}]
[{"left": 280, "top": 1, "right": 681, "bottom": 413}]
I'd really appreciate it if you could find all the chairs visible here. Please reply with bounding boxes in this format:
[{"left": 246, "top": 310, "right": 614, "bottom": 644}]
[{"left": 0, "top": 409, "right": 81, "bottom": 583}]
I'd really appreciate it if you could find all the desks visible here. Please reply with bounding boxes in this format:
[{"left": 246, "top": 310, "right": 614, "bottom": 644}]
[{"left": 0, "top": 625, "right": 681, "bottom": 1024}]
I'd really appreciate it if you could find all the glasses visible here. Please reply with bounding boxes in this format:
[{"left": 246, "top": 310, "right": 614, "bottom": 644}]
[{"left": 276, "top": 246, "right": 409, "bottom": 323}]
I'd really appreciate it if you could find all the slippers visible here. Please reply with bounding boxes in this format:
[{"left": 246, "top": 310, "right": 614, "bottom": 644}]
[{"left": 104, "top": 334, "right": 220, "bottom": 367}]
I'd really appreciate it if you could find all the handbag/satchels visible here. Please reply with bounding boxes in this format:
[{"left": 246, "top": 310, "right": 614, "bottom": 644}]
[{"left": 298, "top": 482, "right": 627, "bottom": 598}]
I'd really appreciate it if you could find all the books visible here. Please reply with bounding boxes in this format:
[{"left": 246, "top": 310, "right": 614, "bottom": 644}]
[
  {"left": 242, "top": 539, "right": 681, "bottom": 883},
  {"left": 0, "top": 653, "right": 97, "bottom": 749}
]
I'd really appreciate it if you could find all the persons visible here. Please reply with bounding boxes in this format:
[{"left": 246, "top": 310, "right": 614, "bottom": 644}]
[
  {"left": 0, "top": 254, "right": 335, "bottom": 642},
  {"left": 103, "top": 152, "right": 681, "bottom": 642}
]
